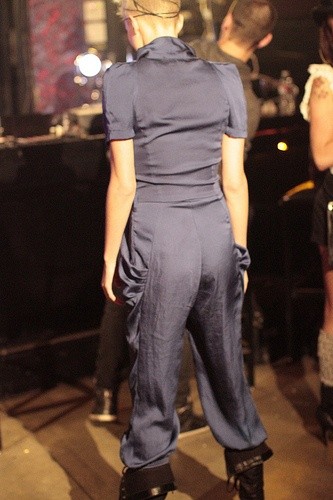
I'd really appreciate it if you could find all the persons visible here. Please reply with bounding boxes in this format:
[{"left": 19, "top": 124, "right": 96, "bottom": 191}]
[
  {"left": 89, "top": 1, "right": 277, "bottom": 439},
  {"left": 298, "top": 18, "right": 333, "bottom": 446},
  {"left": 99, "top": 1, "right": 274, "bottom": 500}
]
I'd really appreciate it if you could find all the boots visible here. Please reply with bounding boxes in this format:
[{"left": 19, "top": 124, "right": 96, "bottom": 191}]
[
  {"left": 317, "top": 382, "right": 333, "bottom": 446},
  {"left": 224, "top": 442, "right": 273, "bottom": 500},
  {"left": 119, "top": 462, "right": 178, "bottom": 500}
]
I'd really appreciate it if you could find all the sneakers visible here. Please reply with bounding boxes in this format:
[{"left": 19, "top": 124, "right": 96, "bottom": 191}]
[
  {"left": 175, "top": 403, "right": 210, "bottom": 439},
  {"left": 88, "top": 389, "right": 118, "bottom": 422}
]
